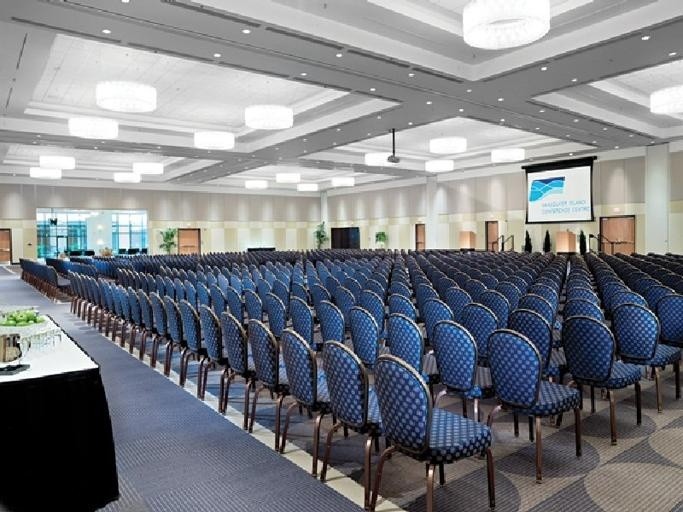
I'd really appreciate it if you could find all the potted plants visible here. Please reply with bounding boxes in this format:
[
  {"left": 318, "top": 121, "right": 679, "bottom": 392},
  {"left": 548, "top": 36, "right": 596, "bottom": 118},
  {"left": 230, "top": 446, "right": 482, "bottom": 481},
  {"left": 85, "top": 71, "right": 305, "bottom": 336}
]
[{"left": 375, "top": 232, "right": 387, "bottom": 249}]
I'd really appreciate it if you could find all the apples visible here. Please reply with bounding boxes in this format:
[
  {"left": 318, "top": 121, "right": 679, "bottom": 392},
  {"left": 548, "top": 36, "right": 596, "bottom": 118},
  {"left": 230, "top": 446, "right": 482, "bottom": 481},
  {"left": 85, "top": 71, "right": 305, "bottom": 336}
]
[{"left": 0, "top": 309, "right": 45, "bottom": 326}]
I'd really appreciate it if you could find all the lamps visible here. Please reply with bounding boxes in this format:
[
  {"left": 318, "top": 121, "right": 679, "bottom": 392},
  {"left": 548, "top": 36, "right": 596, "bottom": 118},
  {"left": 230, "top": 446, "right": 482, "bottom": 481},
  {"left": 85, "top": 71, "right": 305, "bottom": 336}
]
[
  {"left": 245, "top": 105, "right": 294, "bottom": 131},
  {"left": 114, "top": 172, "right": 141, "bottom": 184},
  {"left": 31, "top": 166, "right": 62, "bottom": 180},
  {"left": 193, "top": 130, "right": 235, "bottom": 150},
  {"left": 332, "top": 176, "right": 354, "bottom": 187},
  {"left": 364, "top": 152, "right": 393, "bottom": 167},
  {"left": 68, "top": 115, "right": 120, "bottom": 141},
  {"left": 297, "top": 183, "right": 319, "bottom": 192},
  {"left": 387, "top": 128, "right": 400, "bottom": 163},
  {"left": 39, "top": 155, "right": 75, "bottom": 170},
  {"left": 95, "top": 81, "right": 157, "bottom": 113},
  {"left": 424, "top": 159, "right": 455, "bottom": 174},
  {"left": 463, "top": 0, "right": 552, "bottom": 50},
  {"left": 491, "top": 148, "right": 526, "bottom": 164},
  {"left": 245, "top": 180, "right": 269, "bottom": 190},
  {"left": 275, "top": 172, "right": 301, "bottom": 184},
  {"left": 132, "top": 162, "right": 164, "bottom": 175},
  {"left": 429, "top": 136, "right": 467, "bottom": 155},
  {"left": 648, "top": 83, "right": 683, "bottom": 115}
]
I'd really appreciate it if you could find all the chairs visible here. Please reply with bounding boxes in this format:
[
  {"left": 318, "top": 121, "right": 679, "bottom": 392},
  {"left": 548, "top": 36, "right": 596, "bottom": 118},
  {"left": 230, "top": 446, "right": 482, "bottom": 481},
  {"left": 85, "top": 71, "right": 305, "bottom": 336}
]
[
  {"left": 200, "top": 303, "right": 247, "bottom": 413},
  {"left": 610, "top": 302, "right": 683, "bottom": 413},
  {"left": 555, "top": 314, "right": 643, "bottom": 446},
  {"left": 432, "top": 320, "right": 519, "bottom": 438},
  {"left": 222, "top": 311, "right": 274, "bottom": 430},
  {"left": 370, "top": 353, "right": 496, "bottom": 511},
  {"left": 279, "top": 328, "right": 349, "bottom": 478},
  {"left": 248, "top": 318, "right": 313, "bottom": 453},
  {"left": 481, "top": 329, "right": 583, "bottom": 484},
  {"left": 19, "top": 249, "right": 683, "bottom": 399},
  {"left": 320, "top": 339, "right": 393, "bottom": 510}
]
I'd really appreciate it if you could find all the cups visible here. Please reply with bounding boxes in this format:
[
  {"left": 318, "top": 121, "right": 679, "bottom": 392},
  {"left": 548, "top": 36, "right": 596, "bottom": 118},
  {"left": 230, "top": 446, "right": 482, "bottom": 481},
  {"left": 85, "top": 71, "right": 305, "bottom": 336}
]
[
  {"left": 0, "top": 334, "right": 30, "bottom": 363},
  {"left": 22, "top": 328, "right": 62, "bottom": 351}
]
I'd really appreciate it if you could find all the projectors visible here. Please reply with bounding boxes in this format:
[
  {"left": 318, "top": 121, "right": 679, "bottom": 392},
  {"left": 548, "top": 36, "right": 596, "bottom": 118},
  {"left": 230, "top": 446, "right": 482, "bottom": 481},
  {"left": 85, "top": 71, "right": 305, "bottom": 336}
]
[{"left": 388, "top": 156, "right": 400, "bottom": 163}]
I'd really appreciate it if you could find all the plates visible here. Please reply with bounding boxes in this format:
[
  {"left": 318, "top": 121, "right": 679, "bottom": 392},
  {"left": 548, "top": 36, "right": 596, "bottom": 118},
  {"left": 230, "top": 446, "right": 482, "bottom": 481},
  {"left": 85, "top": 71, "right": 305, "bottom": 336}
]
[{"left": 0, "top": 321, "right": 49, "bottom": 330}]
